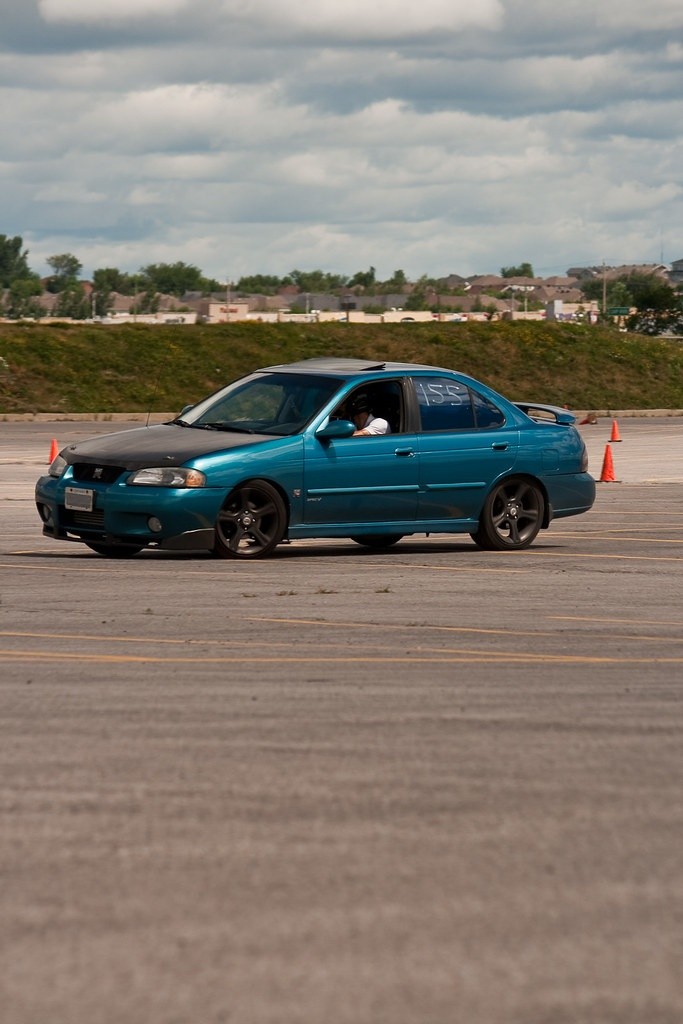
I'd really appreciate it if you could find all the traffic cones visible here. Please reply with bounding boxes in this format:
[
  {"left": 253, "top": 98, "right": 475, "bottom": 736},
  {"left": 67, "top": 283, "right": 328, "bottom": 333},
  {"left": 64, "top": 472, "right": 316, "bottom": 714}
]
[
  {"left": 578, "top": 413, "right": 597, "bottom": 425},
  {"left": 563, "top": 405, "right": 568, "bottom": 409},
  {"left": 608, "top": 420, "right": 623, "bottom": 442},
  {"left": 49, "top": 439, "right": 58, "bottom": 464},
  {"left": 595, "top": 444, "right": 622, "bottom": 483}
]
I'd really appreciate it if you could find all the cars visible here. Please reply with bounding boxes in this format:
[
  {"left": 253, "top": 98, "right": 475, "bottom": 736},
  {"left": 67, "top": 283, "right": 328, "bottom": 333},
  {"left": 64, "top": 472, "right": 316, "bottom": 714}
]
[{"left": 35, "top": 358, "right": 596, "bottom": 560}]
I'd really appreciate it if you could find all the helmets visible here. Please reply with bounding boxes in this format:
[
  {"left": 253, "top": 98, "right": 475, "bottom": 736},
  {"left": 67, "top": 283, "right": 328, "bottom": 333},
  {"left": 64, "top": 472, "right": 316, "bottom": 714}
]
[{"left": 342, "top": 392, "right": 372, "bottom": 415}]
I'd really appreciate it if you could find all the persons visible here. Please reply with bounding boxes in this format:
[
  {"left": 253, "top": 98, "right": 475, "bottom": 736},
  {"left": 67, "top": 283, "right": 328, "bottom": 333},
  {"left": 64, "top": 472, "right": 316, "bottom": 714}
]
[{"left": 338, "top": 388, "right": 391, "bottom": 436}]
